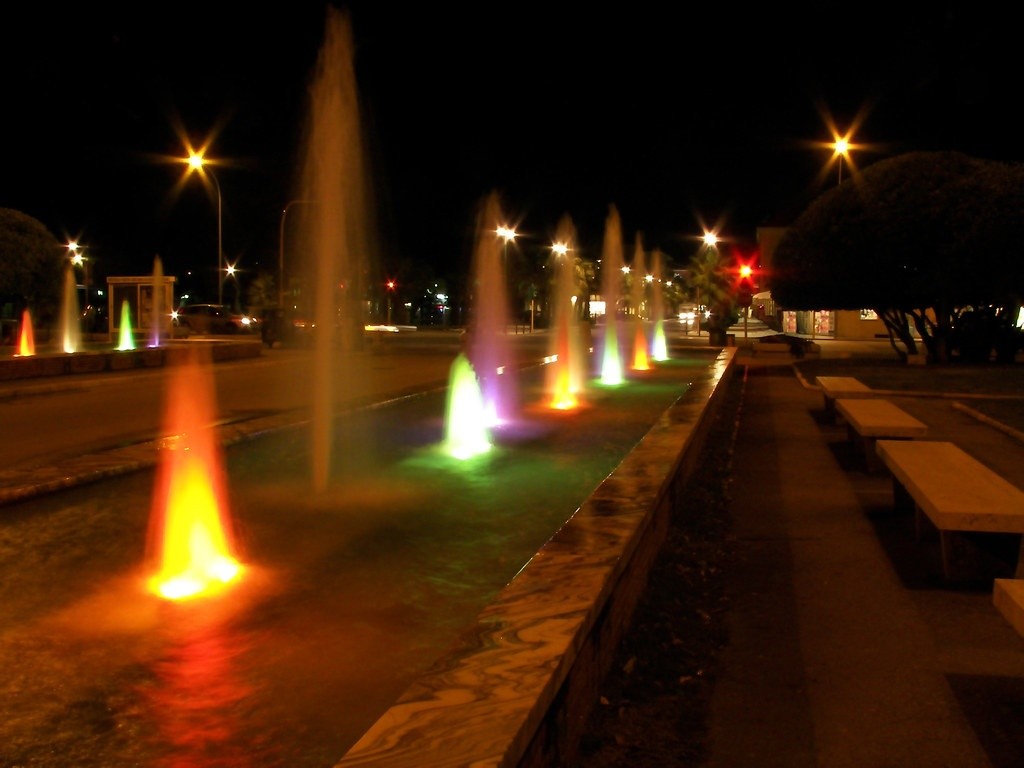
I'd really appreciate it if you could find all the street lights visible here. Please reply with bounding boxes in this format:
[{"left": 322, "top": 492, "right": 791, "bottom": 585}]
[
  {"left": 188, "top": 155, "right": 223, "bottom": 305},
  {"left": 833, "top": 137, "right": 850, "bottom": 183},
  {"left": 496, "top": 223, "right": 515, "bottom": 295}
]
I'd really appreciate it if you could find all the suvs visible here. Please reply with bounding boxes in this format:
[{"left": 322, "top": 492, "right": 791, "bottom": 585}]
[{"left": 262, "top": 299, "right": 363, "bottom": 352}]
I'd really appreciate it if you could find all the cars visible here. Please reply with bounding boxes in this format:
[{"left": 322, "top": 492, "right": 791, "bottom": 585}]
[
  {"left": 174, "top": 304, "right": 258, "bottom": 336},
  {"left": 738, "top": 333, "right": 820, "bottom": 355}
]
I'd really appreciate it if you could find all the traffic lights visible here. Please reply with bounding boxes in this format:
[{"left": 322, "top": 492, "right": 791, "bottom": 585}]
[
  {"left": 739, "top": 262, "right": 755, "bottom": 310},
  {"left": 387, "top": 276, "right": 395, "bottom": 294}
]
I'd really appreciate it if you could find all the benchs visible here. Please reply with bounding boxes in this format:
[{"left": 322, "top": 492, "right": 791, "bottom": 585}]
[
  {"left": 836, "top": 399, "right": 929, "bottom": 476},
  {"left": 990, "top": 577, "right": 1024, "bottom": 638},
  {"left": 874, "top": 440, "right": 1024, "bottom": 593},
  {"left": 815, "top": 376, "right": 877, "bottom": 424}
]
